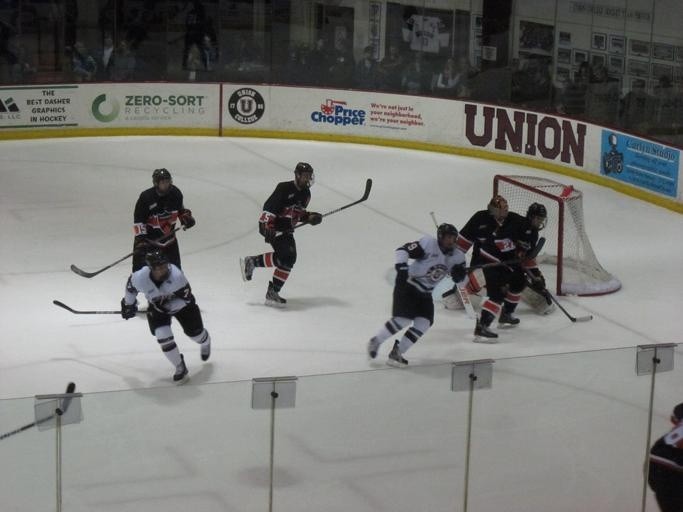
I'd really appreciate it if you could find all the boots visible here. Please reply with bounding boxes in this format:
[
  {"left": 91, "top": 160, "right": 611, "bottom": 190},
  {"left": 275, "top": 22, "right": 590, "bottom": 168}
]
[
  {"left": 368, "top": 336, "right": 380, "bottom": 359},
  {"left": 265, "top": 280, "right": 287, "bottom": 304},
  {"left": 498, "top": 306, "right": 521, "bottom": 324},
  {"left": 389, "top": 340, "right": 409, "bottom": 364},
  {"left": 244, "top": 254, "right": 258, "bottom": 281},
  {"left": 172, "top": 353, "right": 188, "bottom": 380},
  {"left": 474, "top": 317, "right": 498, "bottom": 338},
  {"left": 197, "top": 330, "right": 211, "bottom": 361}
]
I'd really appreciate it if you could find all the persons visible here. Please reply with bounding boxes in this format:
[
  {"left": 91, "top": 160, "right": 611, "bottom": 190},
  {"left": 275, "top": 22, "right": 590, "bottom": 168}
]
[
  {"left": 473, "top": 202, "right": 547, "bottom": 338},
  {"left": 647, "top": 402, "right": 683, "bottom": 512},
  {"left": 120, "top": 248, "right": 211, "bottom": 381},
  {"left": 242, "top": 162, "right": 323, "bottom": 304},
  {"left": 131, "top": 167, "right": 196, "bottom": 273},
  {"left": 443, "top": 196, "right": 552, "bottom": 312},
  {"left": 366, "top": 222, "right": 466, "bottom": 364}
]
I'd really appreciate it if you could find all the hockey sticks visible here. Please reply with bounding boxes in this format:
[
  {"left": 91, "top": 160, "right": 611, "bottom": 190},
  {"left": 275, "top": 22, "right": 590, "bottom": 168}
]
[
  {"left": 70, "top": 224, "right": 185, "bottom": 278},
  {"left": 410, "top": 237, "right": 546, "bottom": 280},
  {"left": 2, "top": 383, "right": 75, "bottom": 443},
  {"left": 526, "top": 267, "right": 592, "bottom": 322},
  {"left": 431, "top": 213, "right": 482, "bottom": 319},
  {"left": 274, "top": 179, "right": 372, "bottom": 236},
  {"left": 53, "top": 300, "right": 154, "bottom": 314}
]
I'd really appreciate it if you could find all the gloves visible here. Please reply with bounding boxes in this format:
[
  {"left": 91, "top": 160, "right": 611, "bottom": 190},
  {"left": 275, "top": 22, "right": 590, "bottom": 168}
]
[
  {"left": 451, "top": 264, "right": 466, "bottom": 283},
  {"left": 395, "top": 263, "right": 409, "bottom": 278},
  {"left": 530, "top": 267, "right": 545, "bottom": 290},
  {"left": 120, "top": 297, "right": 139, "bottom": 321},
  {"left": 177, "top": 207, "right": 195, "bottom": 231},
  {"left": 300, "top": 211, "right": 323, "bottom": 226},
  {"left": 146, "top": 305, "right": 171, "bottom": 323}
]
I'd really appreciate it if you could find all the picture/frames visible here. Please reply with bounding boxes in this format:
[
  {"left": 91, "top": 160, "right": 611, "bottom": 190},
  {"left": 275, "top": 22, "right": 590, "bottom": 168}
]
[
  {"left": 469, "top": 12, "right": 500, "bottom": 66},
  {"left": 365, "top": 2, "right": 381, "bottom": 62},
  {"left": 555, "top": 28, "right": 682, "bottom": 113}
]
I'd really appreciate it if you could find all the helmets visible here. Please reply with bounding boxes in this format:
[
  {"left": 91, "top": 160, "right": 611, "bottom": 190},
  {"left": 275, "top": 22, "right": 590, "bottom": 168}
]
[
  {"left": 294, "top": 162, "right": 314, "bottom": 182},
  {"left": 144, "top": 250, "right": 171, "bottom": 271},
  {"left": 526, "top": 203, "right": 548, "bottom": 231},
  {"left": 152, "top": 168, "right": 173, "bottom": 187},
  {"left": 486, "top": 194, "right": 510, "bottom": 218},
  {"left": 436, "top": 224, "right": 459, "bottom": 246}
]
